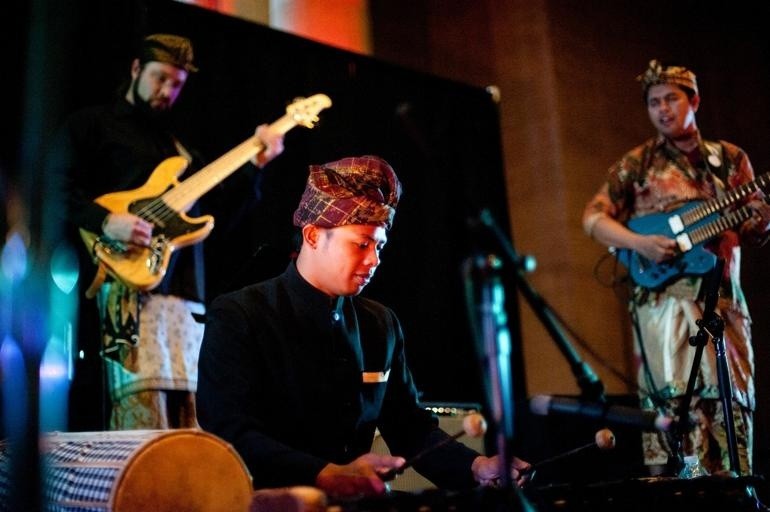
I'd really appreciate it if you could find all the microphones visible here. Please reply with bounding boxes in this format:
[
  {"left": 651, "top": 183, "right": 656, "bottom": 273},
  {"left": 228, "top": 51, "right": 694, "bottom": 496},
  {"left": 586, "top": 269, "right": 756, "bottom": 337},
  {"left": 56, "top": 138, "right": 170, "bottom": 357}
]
[
  {"left": 396, "top": 80, "right": 502, "bottom": 131},
  {"left": 530, "top": 388, "right": 678, "bottom": 433}
]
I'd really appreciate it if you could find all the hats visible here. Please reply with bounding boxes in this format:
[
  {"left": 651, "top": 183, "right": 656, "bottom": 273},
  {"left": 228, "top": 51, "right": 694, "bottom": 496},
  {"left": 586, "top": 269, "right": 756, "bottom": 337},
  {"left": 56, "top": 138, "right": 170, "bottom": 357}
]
[{"left": 143, "top": 34, "right": 198, "bottom": 73}]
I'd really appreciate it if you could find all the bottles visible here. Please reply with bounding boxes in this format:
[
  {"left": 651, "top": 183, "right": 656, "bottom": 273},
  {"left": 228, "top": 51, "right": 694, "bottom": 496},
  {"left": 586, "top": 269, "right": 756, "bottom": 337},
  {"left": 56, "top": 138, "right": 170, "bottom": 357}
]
[{"left": 680, "top": 455, "right": 706, "bottom": 478}]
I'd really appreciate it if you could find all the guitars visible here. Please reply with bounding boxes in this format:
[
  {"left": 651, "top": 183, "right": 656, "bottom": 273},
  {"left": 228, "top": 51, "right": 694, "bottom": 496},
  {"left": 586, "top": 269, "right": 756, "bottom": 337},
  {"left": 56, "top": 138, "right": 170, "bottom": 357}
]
[
  {"left": 81, "top": 93, "right": 332, "bottom": 291},
  {"left": 608, "top": 169, "right": 769, "bottom": 289}
]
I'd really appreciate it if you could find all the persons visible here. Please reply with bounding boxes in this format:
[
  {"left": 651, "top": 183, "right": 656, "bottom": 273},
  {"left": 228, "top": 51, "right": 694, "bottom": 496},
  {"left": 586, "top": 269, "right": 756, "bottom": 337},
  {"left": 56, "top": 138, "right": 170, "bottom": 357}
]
[
  {"left": 61, "top": 35, "right": 286, "bottom": 430},
  {"left": 582, "top": 60, "right": 770, "bottom": 491},
  {"left": 194, "top": 155, "right": 532, "bottom": 512}
]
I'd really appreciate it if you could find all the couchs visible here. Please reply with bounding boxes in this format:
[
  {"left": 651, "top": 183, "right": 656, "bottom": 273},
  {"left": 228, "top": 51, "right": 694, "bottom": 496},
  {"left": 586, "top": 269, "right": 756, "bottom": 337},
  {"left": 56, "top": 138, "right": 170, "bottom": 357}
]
[{"left": 29, "top": 427, "right": 345, "bottom": 512}]
